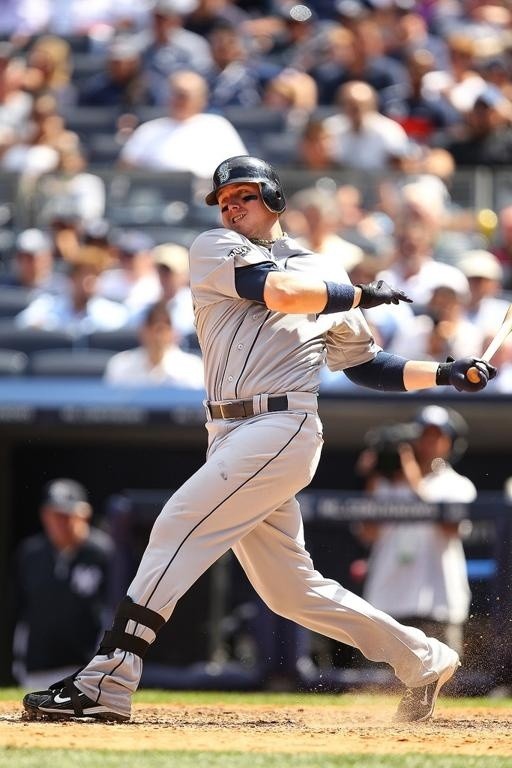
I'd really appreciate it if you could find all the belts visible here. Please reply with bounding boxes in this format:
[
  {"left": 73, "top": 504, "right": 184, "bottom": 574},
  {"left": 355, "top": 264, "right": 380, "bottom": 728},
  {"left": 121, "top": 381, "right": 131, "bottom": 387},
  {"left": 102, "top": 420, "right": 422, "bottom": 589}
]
[{"left": 209, "top": 396, "right": 288, "bottom": 420}]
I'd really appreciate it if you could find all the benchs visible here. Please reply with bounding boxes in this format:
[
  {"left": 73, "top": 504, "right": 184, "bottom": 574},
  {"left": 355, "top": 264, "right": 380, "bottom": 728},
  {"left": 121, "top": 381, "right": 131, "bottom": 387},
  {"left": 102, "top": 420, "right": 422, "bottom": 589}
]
[{"left": 0, "top": 32, "right": 512, "bottom": 386}]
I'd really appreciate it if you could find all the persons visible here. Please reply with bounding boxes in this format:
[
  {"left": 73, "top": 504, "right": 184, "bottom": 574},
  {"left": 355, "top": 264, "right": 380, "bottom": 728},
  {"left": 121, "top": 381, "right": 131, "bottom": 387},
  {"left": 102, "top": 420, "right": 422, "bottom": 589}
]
[
  {"left": 9, "top": 479, "right": 117, "bottom": 687},
  {"left": 22, "top": 157, "right": 498, "bottom": 725},
  {"left": 0, "top": 0, "right": 511, "bottom": 387},
  {"left": 354, "top": 400, "right": 479, "bottom": 659}
]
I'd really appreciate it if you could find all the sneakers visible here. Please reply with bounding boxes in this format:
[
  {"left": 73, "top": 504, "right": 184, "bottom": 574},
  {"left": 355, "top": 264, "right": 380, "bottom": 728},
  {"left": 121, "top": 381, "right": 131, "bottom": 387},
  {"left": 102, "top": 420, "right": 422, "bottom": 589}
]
[
  {"left": 24, "top": 678, "right": 130, "bottom": 723},
  {"left": 392, "top": 651, "right": 460, "bottom": 723}
]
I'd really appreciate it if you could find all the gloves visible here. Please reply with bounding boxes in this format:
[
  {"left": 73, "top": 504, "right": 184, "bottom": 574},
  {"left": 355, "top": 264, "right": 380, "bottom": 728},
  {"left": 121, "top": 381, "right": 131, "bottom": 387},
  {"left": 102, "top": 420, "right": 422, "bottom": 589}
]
[
  {"left": 437, "top": 356, "right": 498, "bottom": 393},
  {"left": 355, "top": 281, "right": 412, "bottom": 308}
]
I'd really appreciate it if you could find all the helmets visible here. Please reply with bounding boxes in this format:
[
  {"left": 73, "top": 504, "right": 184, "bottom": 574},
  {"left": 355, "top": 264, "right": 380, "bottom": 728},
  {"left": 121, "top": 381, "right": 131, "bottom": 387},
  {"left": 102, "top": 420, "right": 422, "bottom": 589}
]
[{"left": 205, "top": 156, "right": 286, "bottom": 214}]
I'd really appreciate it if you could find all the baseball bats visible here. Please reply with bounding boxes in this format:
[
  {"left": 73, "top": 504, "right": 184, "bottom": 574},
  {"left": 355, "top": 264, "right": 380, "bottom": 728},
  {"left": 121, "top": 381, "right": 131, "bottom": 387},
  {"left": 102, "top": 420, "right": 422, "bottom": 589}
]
[{"left": 467, "top": 303, "right": 512, "bottom": 384}]
